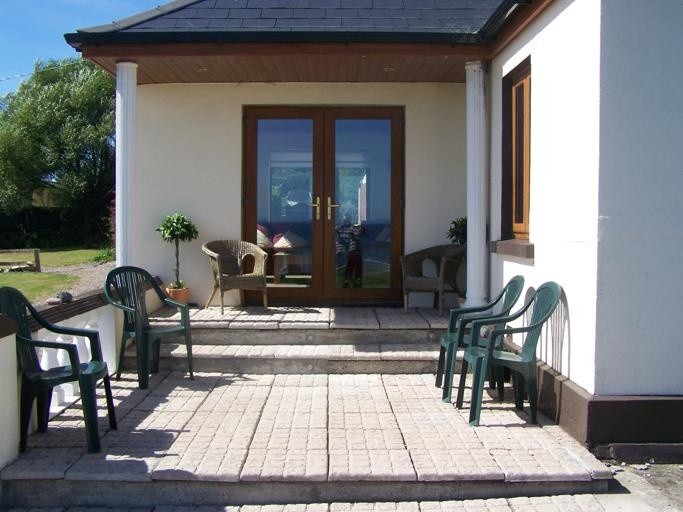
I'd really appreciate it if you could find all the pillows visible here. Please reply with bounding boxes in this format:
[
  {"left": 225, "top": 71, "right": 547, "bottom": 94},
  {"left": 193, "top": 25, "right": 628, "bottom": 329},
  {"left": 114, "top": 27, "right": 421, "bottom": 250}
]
[{"left": 257, "top": 230, "right": 309, "bottom": 253}]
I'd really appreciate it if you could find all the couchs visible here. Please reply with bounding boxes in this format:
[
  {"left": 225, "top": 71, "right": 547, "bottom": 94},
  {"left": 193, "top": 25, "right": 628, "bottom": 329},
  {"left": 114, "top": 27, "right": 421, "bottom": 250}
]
[{"left": 256, "top": 221, "right": 312, "bottom": 274}]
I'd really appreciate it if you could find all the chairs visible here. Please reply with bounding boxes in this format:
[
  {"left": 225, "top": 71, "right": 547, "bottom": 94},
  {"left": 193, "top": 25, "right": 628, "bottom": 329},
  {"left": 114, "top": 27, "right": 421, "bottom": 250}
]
[
  {"left": 104, "top": 265, "right": 194, "bottom": 392},
  {"left": 436, "top": 273, "right": 562, "bottom": 428},
  {"left": 397, "top": 241, "right": 466, "bottom": 318},
  {"left": 2, "top": 285, "right": 121, "bottom": 453},
  {"left": 202, "top": 238, "right": 269, "bottom": 317}
]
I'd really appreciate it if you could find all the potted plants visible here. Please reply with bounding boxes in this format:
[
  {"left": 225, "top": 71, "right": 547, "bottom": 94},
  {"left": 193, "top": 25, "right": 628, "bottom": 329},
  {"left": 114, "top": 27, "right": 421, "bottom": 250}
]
[{"left": 154, "top": 211, "right": 199, "bottom": 305}]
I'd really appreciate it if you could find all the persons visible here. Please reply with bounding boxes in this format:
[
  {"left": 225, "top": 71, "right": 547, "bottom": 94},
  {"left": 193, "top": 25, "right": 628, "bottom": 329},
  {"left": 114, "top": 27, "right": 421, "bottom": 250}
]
[{"left": 335, "top": 212, "right": 363, "bottom": 288}]
